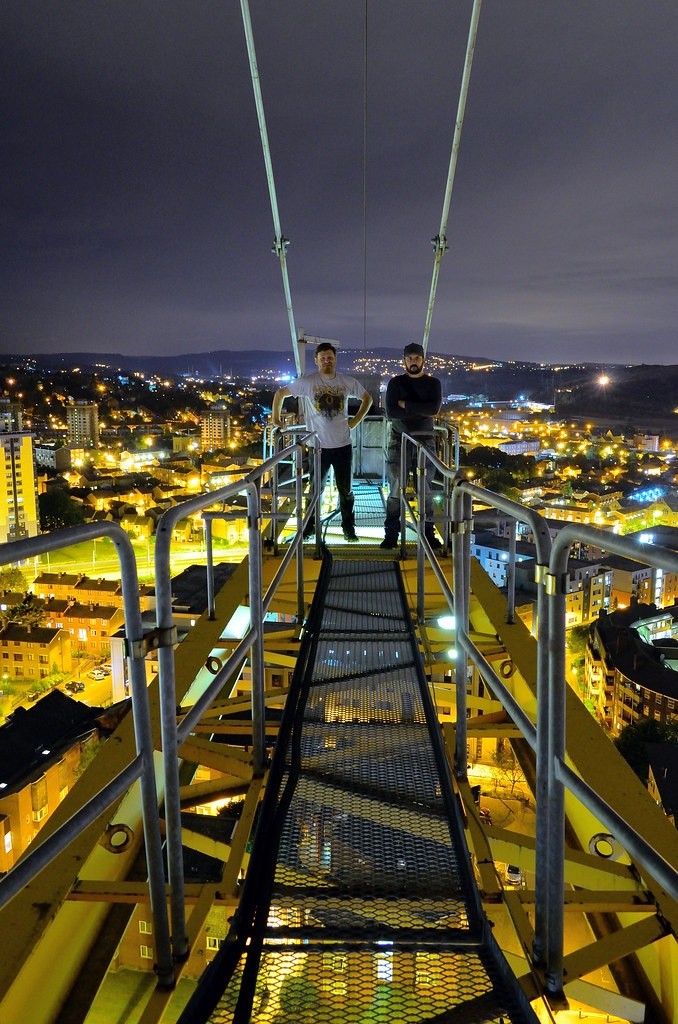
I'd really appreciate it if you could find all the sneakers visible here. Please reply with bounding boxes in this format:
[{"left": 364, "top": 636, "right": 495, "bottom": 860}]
[
  {"left": 425, "top": 537, "right": 443, "bottom": 549},
  {"left": 379, "top": 529, "right": 399, "bottom": 549},
  {"left": 302, "top": 526, "right": 359, "bottom": 542}
]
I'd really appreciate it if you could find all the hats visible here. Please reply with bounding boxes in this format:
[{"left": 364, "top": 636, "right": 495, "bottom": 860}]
[{"left": 403, "top": 343, "right": 424, "bottom": 357}]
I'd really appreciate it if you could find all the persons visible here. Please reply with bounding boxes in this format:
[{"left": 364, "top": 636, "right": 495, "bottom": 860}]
[
  {"left": 376, "top": 344, "right": 443, "bottom": 549},
  {"left": 272, "top": 341, "right": 374, "bottom": 544}
]
